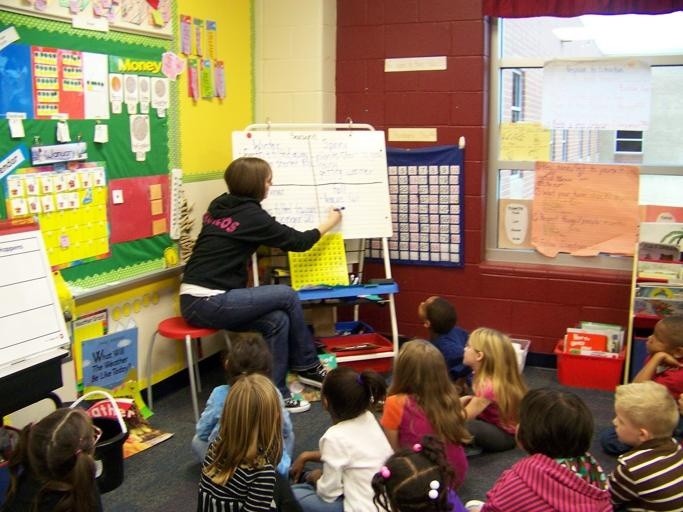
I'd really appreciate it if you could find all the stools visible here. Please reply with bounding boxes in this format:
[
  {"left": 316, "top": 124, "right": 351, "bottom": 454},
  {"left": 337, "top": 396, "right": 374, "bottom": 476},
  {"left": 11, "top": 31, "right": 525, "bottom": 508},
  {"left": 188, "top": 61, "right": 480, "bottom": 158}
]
[{"left": 147, "top": 316, "right": 232, "bottom": 426}]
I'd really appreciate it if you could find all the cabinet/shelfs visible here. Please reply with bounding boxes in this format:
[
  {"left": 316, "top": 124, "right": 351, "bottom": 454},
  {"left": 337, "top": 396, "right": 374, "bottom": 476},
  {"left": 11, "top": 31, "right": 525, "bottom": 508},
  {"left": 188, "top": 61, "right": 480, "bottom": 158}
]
[
  {"left": 239, "top": 125, "right": 398, "bottom": 395},
  {"left": 622, "top": 232, "right": 683, "bottom": 388}
]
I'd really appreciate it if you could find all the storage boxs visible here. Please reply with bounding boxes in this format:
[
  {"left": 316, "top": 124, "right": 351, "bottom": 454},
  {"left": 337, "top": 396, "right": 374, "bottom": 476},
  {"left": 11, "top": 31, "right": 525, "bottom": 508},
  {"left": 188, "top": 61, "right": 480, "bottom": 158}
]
[
  {"left": 308, "top": 321, "right": 375, "bottom": 335},
  {"left": 555, "top": 338, "right": 627, "bottom": 390},
  {"left": 323, "top": 334, "right": 394, "bottom": 376}
]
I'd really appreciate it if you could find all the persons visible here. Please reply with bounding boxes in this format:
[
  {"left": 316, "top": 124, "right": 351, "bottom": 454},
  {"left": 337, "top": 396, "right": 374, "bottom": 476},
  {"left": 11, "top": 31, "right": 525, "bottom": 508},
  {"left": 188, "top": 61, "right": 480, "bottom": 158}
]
[
  {"left": 0, "top": 408, "right": 103, "bottom": 512},
  {"left": 600, "top": 316, "right": 683, "bottom": 459},
  {"left": 380, "top": 339, "right": 468, "bottom": 491},
  {"left": 416, "top": 295, "right": 470, "bottom": 380},
  {"left": 607, "top": 380, "right": 683, "bottom": 512},
  {"left": 459, "top": 327, "right": 527, "bottom": 451},
  {"left": 370, "top": 436, "right": 456, "bottom": 512},
  {"left": 290, "top": 367, "right": 394, "bottom": 512},
  {"left": 197, "top": 373, "right": 284, "bottom": 512},
  {"left": 473, "top": 387, "right": 614, "bottom": 512},
  {"left": 178, "top": 157, "right": 342, "bottom": 414},
  {"left": 191, "top": 333, "right": 295, "bottom": 475}
]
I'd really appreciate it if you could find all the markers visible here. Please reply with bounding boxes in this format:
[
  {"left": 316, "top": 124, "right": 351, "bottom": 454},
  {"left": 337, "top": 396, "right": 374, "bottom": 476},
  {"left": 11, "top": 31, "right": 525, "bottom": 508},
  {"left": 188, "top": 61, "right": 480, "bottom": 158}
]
[{"left": 333, "top": 206, "right": 346, "bottom": 213}]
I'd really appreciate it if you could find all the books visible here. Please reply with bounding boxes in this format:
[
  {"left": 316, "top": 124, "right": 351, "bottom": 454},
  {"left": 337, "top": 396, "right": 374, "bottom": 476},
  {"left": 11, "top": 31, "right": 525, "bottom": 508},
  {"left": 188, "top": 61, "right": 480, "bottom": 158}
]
[
  {"left": 634, "top": 221, "right": 683, "bottom": 316},
  {"left": 562, "top": 320, "right": 624, "bottom": 358}
]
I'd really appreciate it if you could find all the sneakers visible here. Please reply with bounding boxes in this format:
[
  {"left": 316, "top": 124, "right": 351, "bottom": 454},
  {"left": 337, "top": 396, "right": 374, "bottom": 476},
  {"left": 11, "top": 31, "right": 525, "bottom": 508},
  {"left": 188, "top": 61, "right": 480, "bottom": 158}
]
[
  {"left": 284, "top": 398, "right": 311, "bottom": 414},
  {"left": 296, "top": 365, "right": 328, "bottom": 389}
]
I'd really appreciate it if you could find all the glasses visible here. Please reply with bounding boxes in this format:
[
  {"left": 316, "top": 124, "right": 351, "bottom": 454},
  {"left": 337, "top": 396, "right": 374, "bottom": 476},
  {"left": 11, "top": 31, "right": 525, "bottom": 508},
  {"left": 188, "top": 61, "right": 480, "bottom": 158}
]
[{"left": 92, "top": 425, "right": 102, "bottom": 447}]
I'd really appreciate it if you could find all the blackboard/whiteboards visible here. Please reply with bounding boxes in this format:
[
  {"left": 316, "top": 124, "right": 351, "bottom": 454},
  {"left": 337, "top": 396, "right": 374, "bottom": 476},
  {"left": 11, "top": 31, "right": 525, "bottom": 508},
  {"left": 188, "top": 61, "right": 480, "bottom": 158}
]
[{"left": 230, "top": 128, "right": 394, "bottom": 240}]
